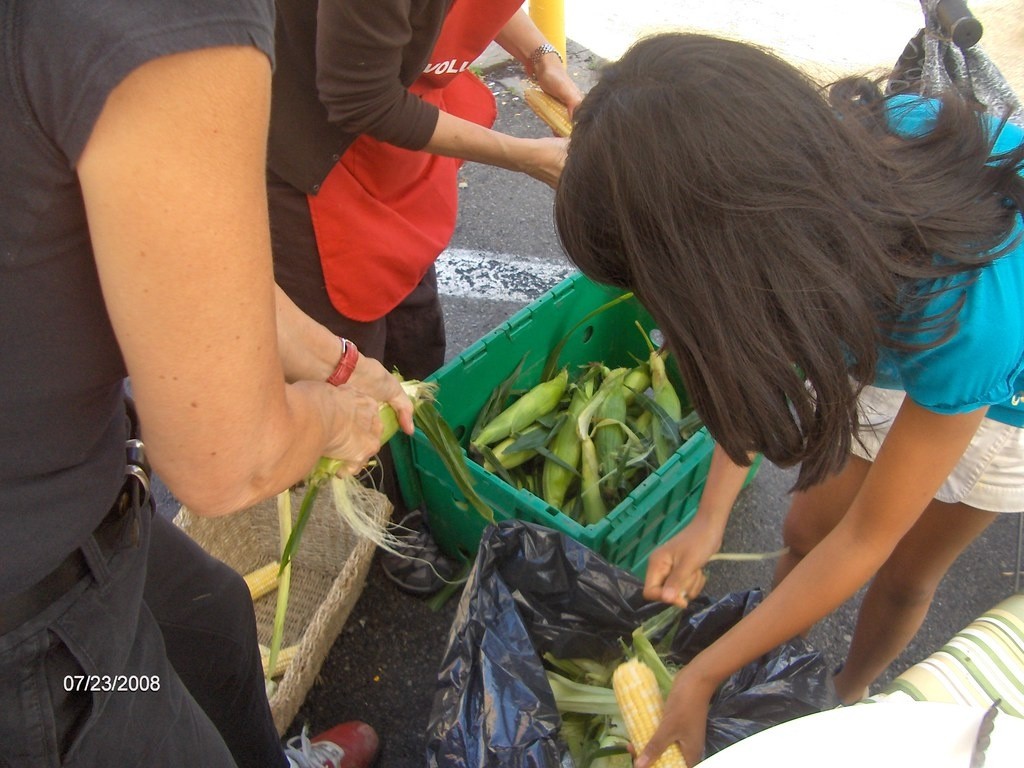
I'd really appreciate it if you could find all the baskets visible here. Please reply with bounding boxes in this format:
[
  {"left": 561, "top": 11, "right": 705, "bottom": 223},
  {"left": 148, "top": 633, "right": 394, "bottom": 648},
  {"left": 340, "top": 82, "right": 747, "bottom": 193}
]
[{"left": 172, "top": 478, "right": 394, "bottom": 743}]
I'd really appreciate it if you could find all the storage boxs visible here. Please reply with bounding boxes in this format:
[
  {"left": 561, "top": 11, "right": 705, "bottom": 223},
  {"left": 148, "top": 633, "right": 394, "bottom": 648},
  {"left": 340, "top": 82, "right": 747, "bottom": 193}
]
[{"left": 391, "top": 269, "right": 808, "bottom": 575}]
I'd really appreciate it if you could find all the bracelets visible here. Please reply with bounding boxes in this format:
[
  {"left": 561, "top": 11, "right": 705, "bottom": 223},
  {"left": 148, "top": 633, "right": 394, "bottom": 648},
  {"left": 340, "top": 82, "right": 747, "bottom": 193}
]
[
  {"left": 526, "top": 44, "right": 563, "bottom": 83},
  {"left": 327, "top": 337, "right": 358, "bottom": 386}
]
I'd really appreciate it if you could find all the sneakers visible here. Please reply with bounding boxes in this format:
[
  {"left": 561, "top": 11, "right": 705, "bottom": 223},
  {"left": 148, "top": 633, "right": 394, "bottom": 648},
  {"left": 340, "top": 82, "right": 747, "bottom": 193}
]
[
  {"left": 284, "top": 719, "right": 379, "bottom": 768},
  {"left": 380, "top": 516, "right": 452, "bottom": 592}
]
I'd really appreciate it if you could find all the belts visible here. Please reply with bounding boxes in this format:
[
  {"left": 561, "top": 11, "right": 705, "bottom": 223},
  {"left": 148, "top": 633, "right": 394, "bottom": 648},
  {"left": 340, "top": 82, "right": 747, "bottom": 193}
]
[{"left": 0, "top": 390, "right": 152, "bottom": 642}]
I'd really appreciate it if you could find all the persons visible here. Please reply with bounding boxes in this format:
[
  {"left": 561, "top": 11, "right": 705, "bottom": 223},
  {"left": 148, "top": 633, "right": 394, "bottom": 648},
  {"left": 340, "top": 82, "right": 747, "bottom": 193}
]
[
  {"left": 0, "top": 0, "right": 414, "bottom": 768},
  {"left": 556, "top": 34, "right": 1024, "bottom": 768},
  {"left": 266, "top": 0, "right": 585, "bottom": 593}
]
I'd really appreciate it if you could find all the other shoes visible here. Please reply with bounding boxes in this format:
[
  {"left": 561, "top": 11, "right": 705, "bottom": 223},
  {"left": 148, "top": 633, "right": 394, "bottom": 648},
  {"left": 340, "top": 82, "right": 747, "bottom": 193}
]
[{"left": 823, "top": 661, "right": 869, "bottom": 709}]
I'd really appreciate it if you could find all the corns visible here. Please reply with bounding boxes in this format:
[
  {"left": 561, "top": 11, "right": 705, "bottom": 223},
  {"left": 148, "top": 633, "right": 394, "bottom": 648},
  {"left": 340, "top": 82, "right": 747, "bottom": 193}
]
[
  {"left": 314, "top": 379, "right": 429, "bottom": 475},
  {"left": 523, "top": 88, "right": 576, "bottom": 139},
  {"left": 469, "top": 350, "right": 689, "bottom": 525},
  {"left": 242, "top": 559, "right": 281, "bottom": 601},
  {"left": 611, "top": 659, "right": 687, "bottom": 768},
  {"left": 258, "top": 641, "right": 303, "bottom": 696}
]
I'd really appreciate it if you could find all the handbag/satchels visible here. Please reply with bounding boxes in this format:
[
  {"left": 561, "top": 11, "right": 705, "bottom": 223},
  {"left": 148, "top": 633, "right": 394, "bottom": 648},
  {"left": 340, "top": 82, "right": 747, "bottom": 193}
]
[{"left": 885, "top": 1, "right": 1024, "bottom": 126}]
